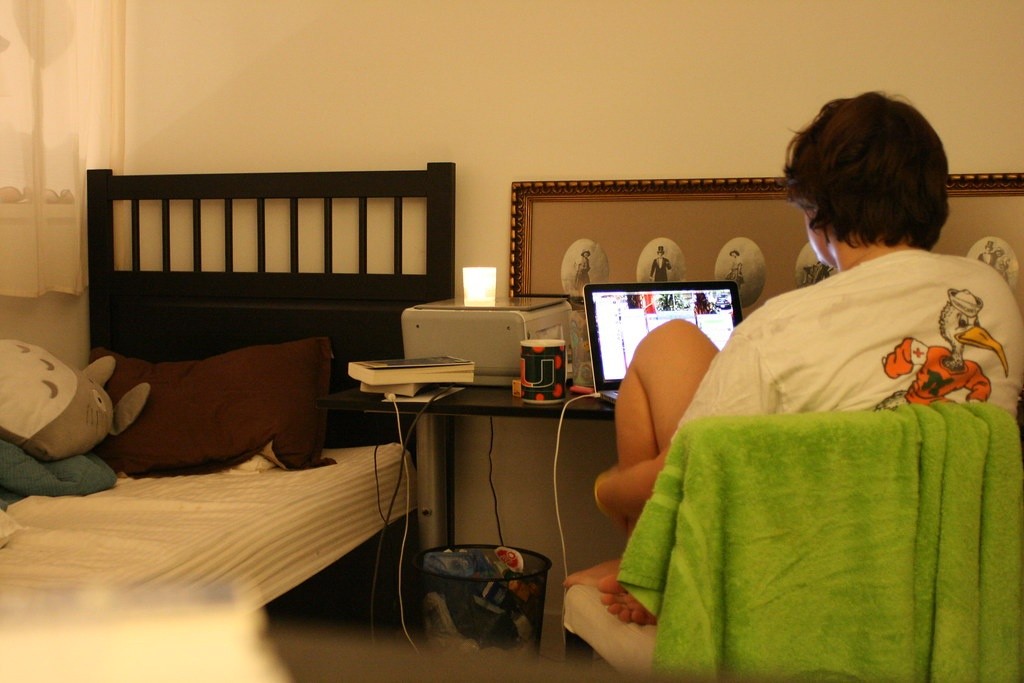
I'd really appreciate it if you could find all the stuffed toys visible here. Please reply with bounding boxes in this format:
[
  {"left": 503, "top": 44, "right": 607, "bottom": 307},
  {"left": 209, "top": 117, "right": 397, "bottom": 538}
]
[{"left": 0, "top": 335, "right": 151, "bottom": 463}]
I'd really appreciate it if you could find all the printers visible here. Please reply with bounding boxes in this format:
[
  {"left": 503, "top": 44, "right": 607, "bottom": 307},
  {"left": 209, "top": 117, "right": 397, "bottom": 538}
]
[{"left": 401, "top": 296, "right": 572, "bottom": 386}]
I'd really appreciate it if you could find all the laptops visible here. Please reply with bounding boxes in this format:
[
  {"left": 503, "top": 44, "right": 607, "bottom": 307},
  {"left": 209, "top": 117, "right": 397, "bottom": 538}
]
[{"left": 582, "top": 280, "right": 743, "bottom": 404}]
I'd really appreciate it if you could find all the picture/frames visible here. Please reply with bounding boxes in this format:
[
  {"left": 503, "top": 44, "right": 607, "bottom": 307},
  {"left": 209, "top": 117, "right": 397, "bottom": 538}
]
[{"left": 505, "top": 171, "right": 1024, "bottom": 385}]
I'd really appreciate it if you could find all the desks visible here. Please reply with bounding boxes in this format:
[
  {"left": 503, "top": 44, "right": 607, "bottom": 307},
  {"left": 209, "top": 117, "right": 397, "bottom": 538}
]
[{"left": 312, "top": 386, "right": 618, "bottom": 655}]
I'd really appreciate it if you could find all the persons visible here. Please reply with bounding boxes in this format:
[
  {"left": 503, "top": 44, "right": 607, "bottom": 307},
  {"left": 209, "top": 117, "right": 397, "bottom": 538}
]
[
  {"left": 561, "top": 93, "right": 1023, "bottom": 628},
  {"left": 978, "top": 240, "right": 1011, "bottom": 283},
  {"left": 574, "top": 249, "right": 590, "bottom": 292},
  {"left": 649, "top": 246, "right": 671, "bottom": 282},
  {"left": 725, "top": 250, "right": 746, "bottom": 290}
]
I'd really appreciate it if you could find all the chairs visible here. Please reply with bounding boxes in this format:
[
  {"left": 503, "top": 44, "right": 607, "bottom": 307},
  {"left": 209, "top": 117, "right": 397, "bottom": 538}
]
[{"left": 562, "top": 401, "right": 1024, "bottom": 683}]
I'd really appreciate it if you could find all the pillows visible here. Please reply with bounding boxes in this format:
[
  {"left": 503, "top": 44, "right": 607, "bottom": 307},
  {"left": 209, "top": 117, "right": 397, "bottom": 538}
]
[
  {"left": 87, "top": 336, "right": 337, "bottom": 476},
  {"left": 0, "top": 338, "right": 152, "bottom": 463}
]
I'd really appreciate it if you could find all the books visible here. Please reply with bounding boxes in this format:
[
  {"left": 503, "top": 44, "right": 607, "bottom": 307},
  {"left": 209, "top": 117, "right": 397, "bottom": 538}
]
[{"left": 348, "top": 355, "right": 476, "bottom": 398}]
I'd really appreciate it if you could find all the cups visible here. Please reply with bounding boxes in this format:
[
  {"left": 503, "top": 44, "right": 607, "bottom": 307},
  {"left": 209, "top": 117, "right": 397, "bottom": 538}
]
[
  {"left": 520, "top": 338, "right": 567, "bottom": 403},
  {"left": 569, "top": 310, "right": 594, "bottom": 388}
]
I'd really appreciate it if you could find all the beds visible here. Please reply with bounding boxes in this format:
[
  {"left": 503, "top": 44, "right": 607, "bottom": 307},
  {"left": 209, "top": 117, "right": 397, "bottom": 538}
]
[{"left": 1, "top": 158, "right": 459, "bottom": 623}]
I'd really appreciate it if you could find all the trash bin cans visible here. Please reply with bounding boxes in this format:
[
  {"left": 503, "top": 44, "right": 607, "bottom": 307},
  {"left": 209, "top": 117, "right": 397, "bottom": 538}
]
[{"left": 412, "top": 543, "right": 552, "bottom": 666}]
